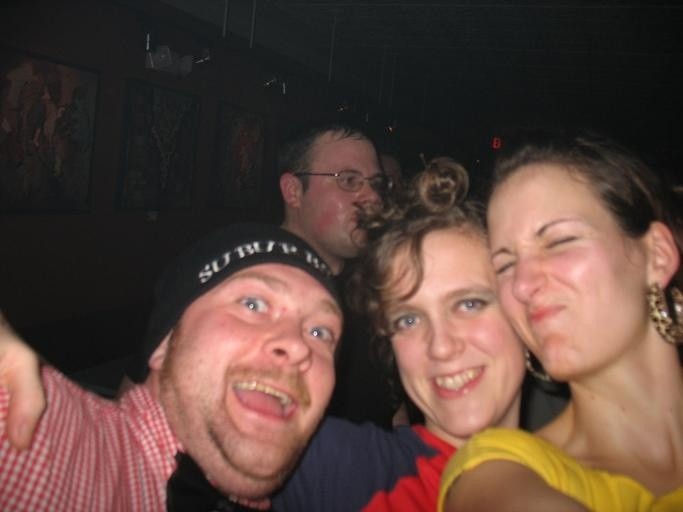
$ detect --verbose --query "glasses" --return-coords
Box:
[293,168,394,196]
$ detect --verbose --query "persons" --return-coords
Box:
[438,127,683,512]
[0,223,344,512]
[272,113,389,280]
[0,156,531,512]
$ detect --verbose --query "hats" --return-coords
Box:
[141,220,346,368]
